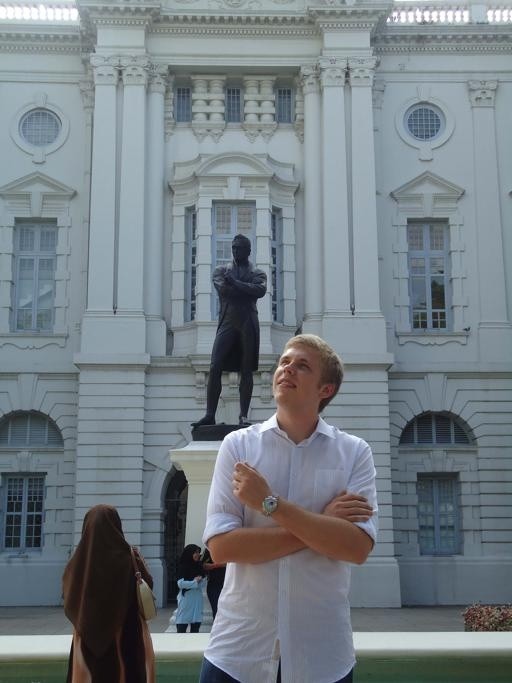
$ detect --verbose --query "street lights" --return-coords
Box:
[128,544,158,623]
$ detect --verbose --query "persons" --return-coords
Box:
[61,504,158,683]
[196,332,378,682]
[190,233,267,426]
[175,544,207,631]
[202,548,227,625]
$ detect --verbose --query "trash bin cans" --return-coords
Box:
[261,491,279,520]
